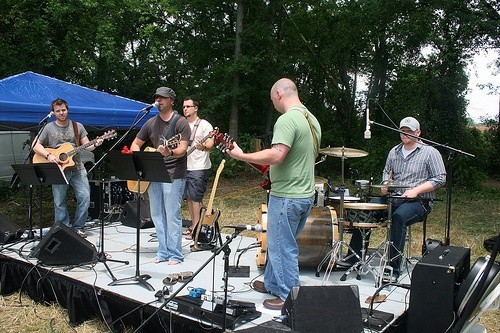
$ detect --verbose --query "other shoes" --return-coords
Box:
[182,228,193,240]
[382,273,398,283]
[335,259,352,268]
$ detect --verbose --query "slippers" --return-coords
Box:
[163,271,194,285]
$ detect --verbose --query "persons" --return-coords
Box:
[331,117,447,287]
[130,87,191,264]
[31,97,105,237]
[170,96,216,254]
[226,78,323,310]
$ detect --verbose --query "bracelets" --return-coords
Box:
[163,147,174,160]
[44,153,50,160]
[94,141,102,148]
[201,145,207,152]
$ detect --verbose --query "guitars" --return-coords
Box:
[32,128,118,172]
[126,132,181,194]
[191,158,226,244]
[214,132,271,190]
[186,126,219,157]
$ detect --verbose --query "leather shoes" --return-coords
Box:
[263,297,285,310]
[253,281,268,293]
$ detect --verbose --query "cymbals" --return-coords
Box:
[318,147,369,158]
[361,184,416,188]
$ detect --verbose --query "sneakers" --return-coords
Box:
[74,228,87,238]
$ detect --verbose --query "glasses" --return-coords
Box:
[155,96,167,99]
[182,106,196,109]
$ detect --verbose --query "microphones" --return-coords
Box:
[142,102,160,110]
[225,224,263,232]
[39,111,54,124]
[364,100,372,139]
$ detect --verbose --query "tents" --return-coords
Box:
[0,70,159,226]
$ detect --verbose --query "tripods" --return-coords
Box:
[63,149,175,292]
[0,121,47,251]
[315,151,415,285]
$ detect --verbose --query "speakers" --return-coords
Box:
[120,199,156,228]
[33,221,96,266]
[0,217,24,244]
[282,285,364,333]
[408,244,471,333]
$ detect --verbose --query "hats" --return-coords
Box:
[153,87,176,98]
[399,117,421,131]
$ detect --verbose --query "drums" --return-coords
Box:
[256,203,340,269]
[314,175,330,207]
[328,196,361,219]
[337,202,389,229]
